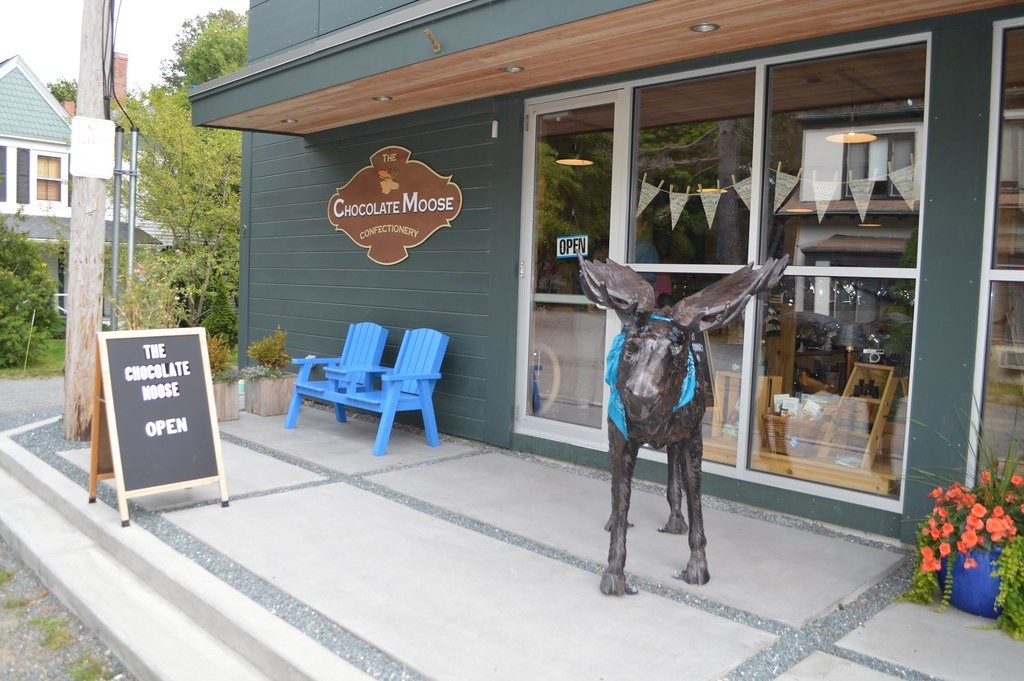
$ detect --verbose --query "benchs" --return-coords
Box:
[283,322,450,456]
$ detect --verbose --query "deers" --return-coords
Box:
[575,252,789,597]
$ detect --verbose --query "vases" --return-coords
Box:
[933,547,1006,621]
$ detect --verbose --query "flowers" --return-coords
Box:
[899,382,1023,640]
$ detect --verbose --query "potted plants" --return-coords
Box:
[241,327,297,418]
[207,329,240,423]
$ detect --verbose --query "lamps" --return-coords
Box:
[555,142,593,166]
[825,122,878,144]
